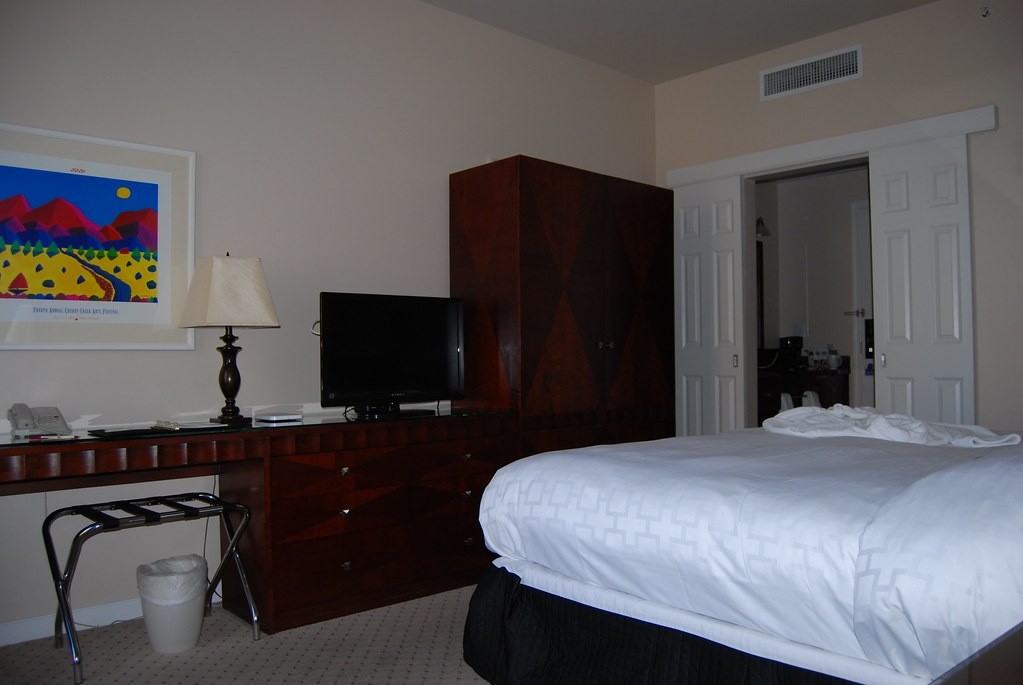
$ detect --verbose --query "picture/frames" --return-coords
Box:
[0,124,195,352]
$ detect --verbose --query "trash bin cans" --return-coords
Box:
[135,554,208,654]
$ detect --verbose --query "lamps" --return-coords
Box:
[176,251,281,422]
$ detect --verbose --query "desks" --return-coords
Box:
[758,365,849,424]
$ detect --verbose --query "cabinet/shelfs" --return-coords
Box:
[0,404,514,638]
[449,153,678,459]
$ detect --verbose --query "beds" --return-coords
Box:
[460,400,1023,684]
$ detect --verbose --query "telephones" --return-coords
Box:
[7,402,72,436]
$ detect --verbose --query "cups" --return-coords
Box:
[829,349,842,370]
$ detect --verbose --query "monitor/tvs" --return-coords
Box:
[319,292,465,422]
